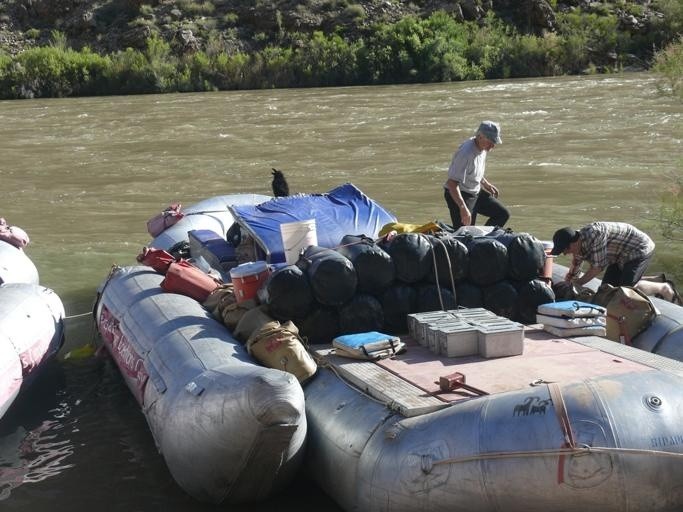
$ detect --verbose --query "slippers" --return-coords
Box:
[656,274,682,306]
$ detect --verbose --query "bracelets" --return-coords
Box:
[458,203,465,210]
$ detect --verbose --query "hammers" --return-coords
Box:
[440,372,490,396]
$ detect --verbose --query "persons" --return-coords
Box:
[442,119,509,232]
[548,218,681,306]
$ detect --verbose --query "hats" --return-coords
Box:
[480,121,503,145]
[551,227,575,255]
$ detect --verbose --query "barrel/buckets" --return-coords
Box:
[540,241,557,287]
[280,219,318,265]
[230,261,271,303]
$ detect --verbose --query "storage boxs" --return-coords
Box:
[406,306,524,359]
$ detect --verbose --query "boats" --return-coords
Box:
[0,216,68,424]
[90,186,681,511]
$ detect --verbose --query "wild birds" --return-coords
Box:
[269,167,290,198]
[225,222,243,248]
